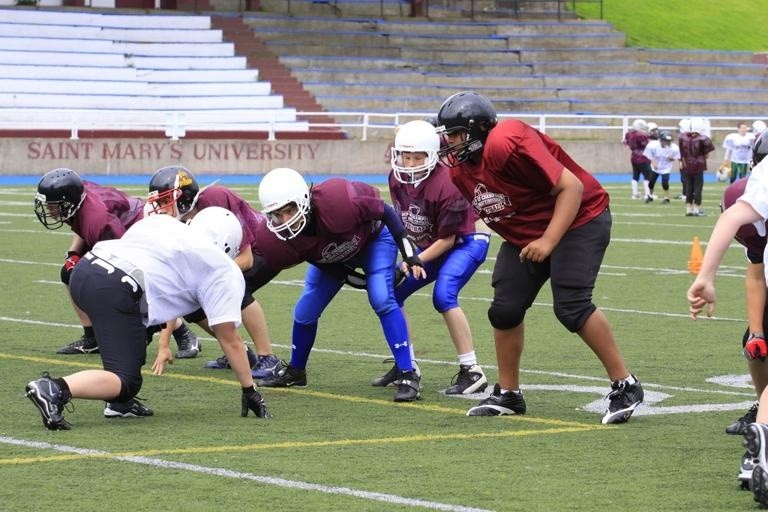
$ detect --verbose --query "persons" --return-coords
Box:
[372,120,491,395]
[34,168,203,359]
[148,166,282,378]
[25,205,275,430]
[726,128,768,435]
[625,117,766,217]
[435,91,644,424]
[686,154,768,506]
[256,168,427,402]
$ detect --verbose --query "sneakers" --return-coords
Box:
[725,402,759,435]
[632,192,640,200]
[250,353,283,379]
[204,345,258,370]
[174,325,202,359]
[370,357,405,388]
[393,370,421,403]
[255,359,309,388]
[734,418,768,506]
[443,363,489,397]
[102,395,155,418]
[685,210,692,217]
[673,194,683,200]
[600,375,645,426]
[693,209,706,216]
[56,333,101,354]
[466,382,527,418]
[663,197,670,204]
[23,371,76,431]
[645,194,658,203]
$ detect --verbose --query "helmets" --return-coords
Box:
[257,167,312,241]
[632,118,648,132]
[660,131,674,146]
[186,206,244,261]
[717,164,732,183]
[678,117,689,132]
[147,165,200,218]
[648,120,658,138]
[390,119,441,185]
[752,133,768,164]
[752,120,766,132]
[437,91,499,165]
[692,119,705,133]
[34,167,87,230]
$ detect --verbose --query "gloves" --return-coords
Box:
[60,248,80,286]
[744,334,768,363]
[240,383,273,420]
